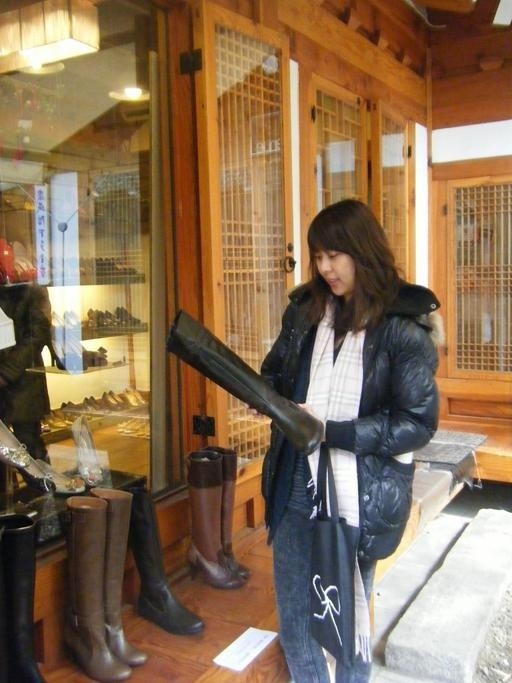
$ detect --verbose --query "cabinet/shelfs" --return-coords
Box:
[41,272,148,448]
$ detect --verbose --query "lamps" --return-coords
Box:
[109,64,153,101]
[0,0,101,72]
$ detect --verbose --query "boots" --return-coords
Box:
[205,445,249,581]
[0,515,48,682]
[186,451,247,589]
[63,495,132,683]
[119,476,204,634]
[89,487,147,666]
[167,309,325,454]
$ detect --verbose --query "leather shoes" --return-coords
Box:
[17,454,55,495]
[81,257,138,277]
[87,307,142,328]
[0,420,30,468]
[39,388,147,433]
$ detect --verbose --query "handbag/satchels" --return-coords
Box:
[308,443,363,668]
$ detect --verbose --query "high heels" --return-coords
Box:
[71,414,105,488]
[36,458,85,494]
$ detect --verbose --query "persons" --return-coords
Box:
[261,201,439,683]
[0,283,52,488]
